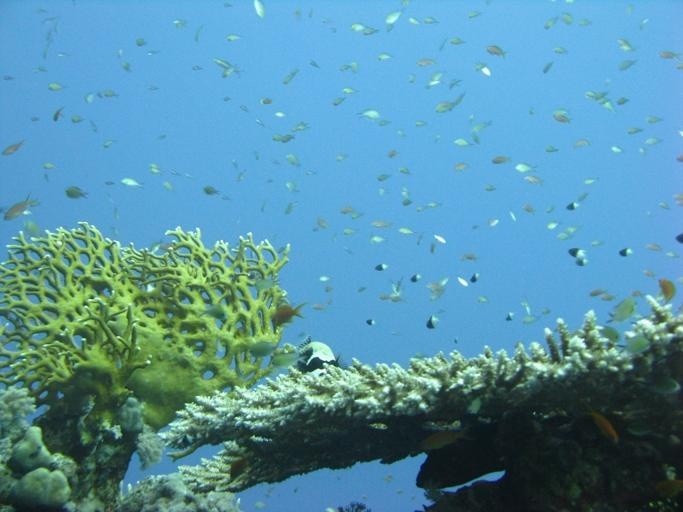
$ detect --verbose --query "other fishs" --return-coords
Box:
[1,0,682,331]
